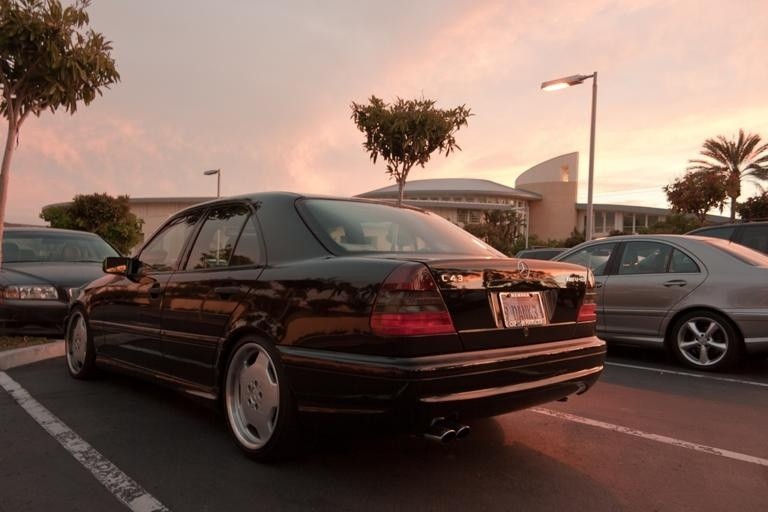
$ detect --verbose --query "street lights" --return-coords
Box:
[539,70,600,242]
[204,168,221,200]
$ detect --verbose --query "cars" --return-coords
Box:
[55,190,610,465]
[515,218,768,374]
[1,224,123,341]
